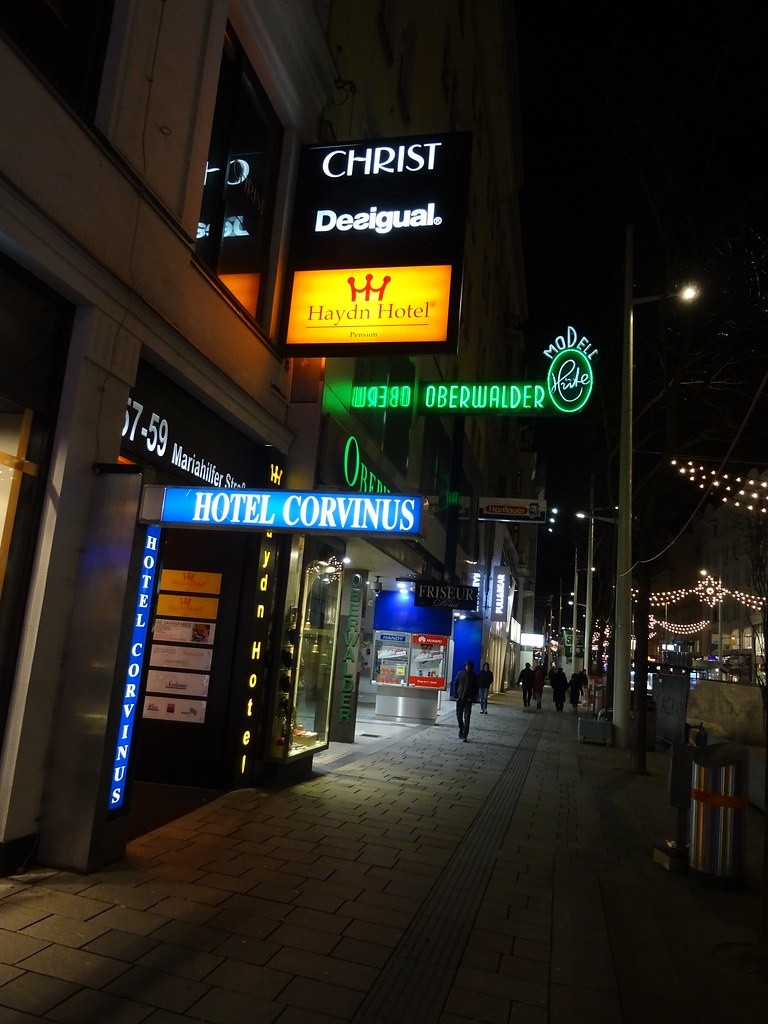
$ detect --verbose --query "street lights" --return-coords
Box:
[610,207,700,746]
[551,470,639,697]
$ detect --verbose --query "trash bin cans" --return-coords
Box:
[687,743,748,876]
[632,698,657,751]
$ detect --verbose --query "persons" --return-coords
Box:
[548,667,556,688]
[551,668,568,712]
[518,663,534,707]
[578,669,587,685]
[453,660,480,742]
[568,673,584,714]
[533,666,545,709]
[477,663,493,714]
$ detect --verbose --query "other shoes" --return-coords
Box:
[480,712,483,714]
[485,712,487,714]
[463,738,467,742]
[459,731,462,738]
